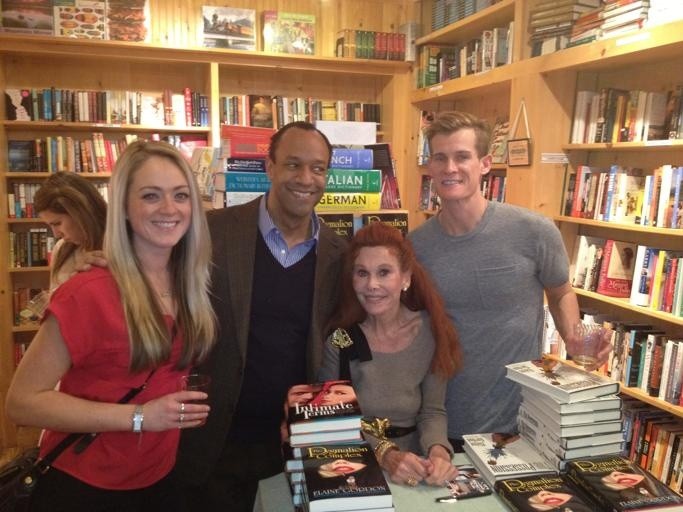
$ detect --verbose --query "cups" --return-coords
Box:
[176,373,211,429]
[573,323,605,369]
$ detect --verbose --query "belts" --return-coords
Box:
[382,425,420,439]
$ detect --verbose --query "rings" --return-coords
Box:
[407,478,416,485]
[179,402,184,412]
[178,413,186,422]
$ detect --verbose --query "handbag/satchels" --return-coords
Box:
[0,445,45,512]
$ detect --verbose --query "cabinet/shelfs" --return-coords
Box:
[412,2,680,505]
[0,2,410,453]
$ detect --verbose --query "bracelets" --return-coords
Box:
[132,404,145,432]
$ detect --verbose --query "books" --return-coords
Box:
[562,87,683,229]
[413,0,681,90]
[457,353,681,511]
[543,234,681,405]
[415,107,514,210]
[12,287,47,369]
[269,379,396,511]
[0,0,417,63]
[6,180,114,268]
[190,93,410,241]
[3,86,211,175]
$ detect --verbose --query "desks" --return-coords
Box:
[258,450,675,509]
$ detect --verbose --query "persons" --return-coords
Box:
[67,121,348,512]
[403,111,613,453]
[5,138,223,510]
[31,170,110,286]
[317,223,463,489]
